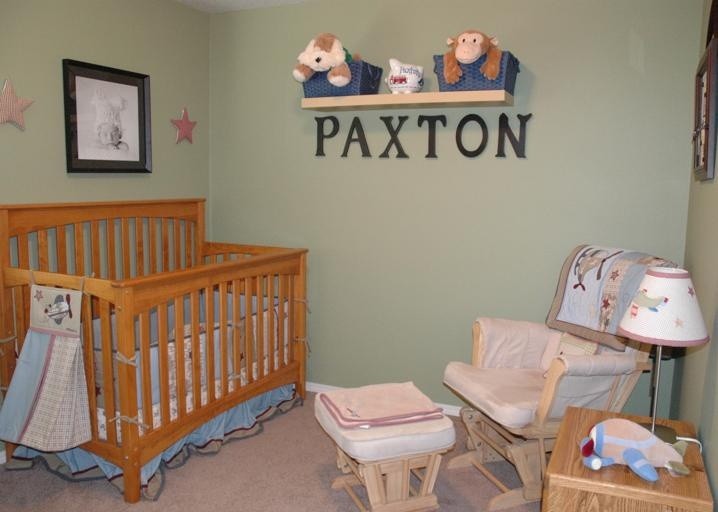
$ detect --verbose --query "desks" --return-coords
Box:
[541,405,718,512]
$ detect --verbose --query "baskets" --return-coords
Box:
[433,51,520,96]
[302,60,382,98]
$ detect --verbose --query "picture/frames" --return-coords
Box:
[690,34,716,183]
[62,57,152,176]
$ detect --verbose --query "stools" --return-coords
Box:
[311,382,455,511]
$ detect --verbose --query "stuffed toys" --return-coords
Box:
[441,29,504,84]
[290,30,362,88]
[576,417,688,483]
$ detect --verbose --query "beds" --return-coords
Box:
[3,197,307,503]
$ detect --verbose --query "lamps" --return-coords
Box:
[618,265,711,445]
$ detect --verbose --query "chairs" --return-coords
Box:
[444,247,680,506]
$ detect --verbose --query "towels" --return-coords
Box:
[319,378,443,432]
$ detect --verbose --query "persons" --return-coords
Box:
[89,89,131,155]
